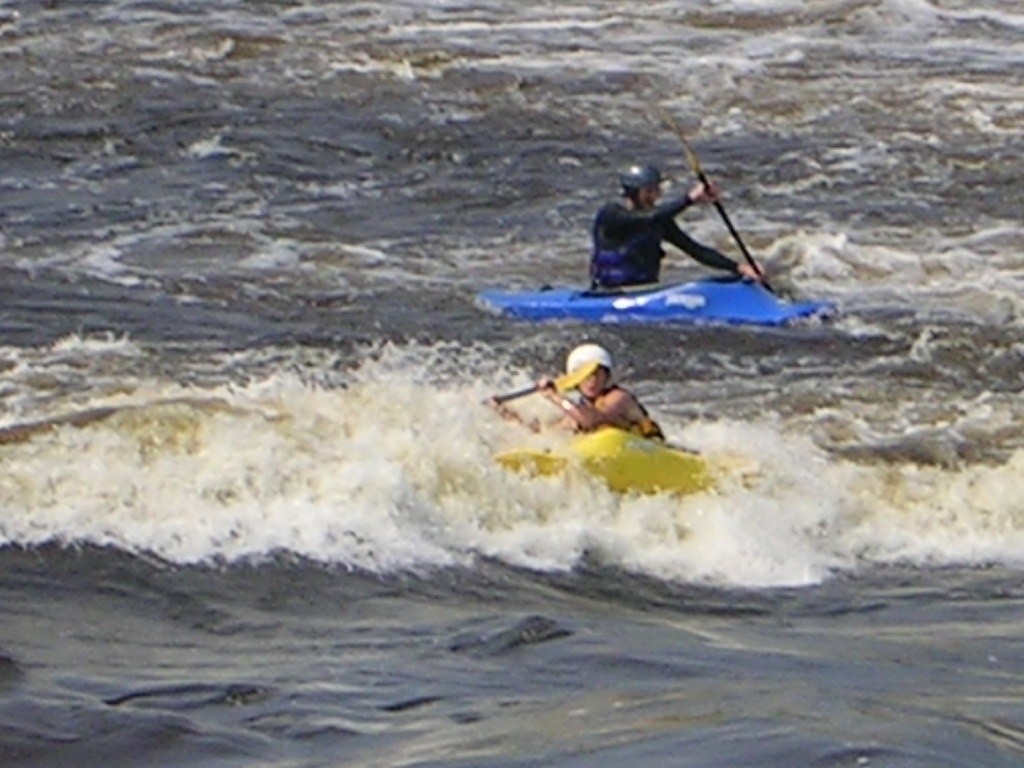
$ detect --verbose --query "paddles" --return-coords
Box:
[477,358,604,405]
[658,102,775,294]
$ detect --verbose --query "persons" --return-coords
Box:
[589,163,766,288]
[488,344,664,444]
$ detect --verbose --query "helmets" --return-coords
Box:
[618,160,662,196]
[566,343,613,376]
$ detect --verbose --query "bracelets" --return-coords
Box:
[562,399,576,413]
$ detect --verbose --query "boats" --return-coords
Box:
[477,272,845,330]
[489,425,723,501]
